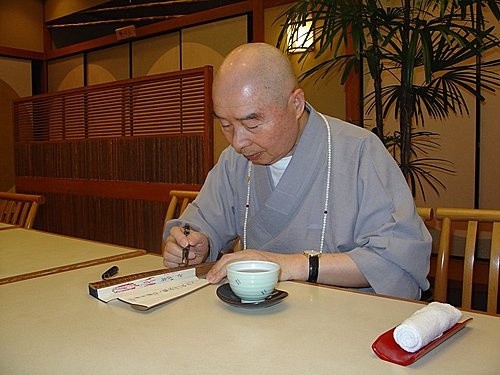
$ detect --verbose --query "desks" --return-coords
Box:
[0,253,500,375]
[1,222,147,284]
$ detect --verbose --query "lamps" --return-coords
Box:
[287,13,315,54]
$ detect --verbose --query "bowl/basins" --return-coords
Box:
[225,260,280,303]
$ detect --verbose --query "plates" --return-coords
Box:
[216,282,289,309]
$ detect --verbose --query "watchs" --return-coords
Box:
[303,248,321,283]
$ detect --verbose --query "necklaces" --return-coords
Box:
[243,111,331,253]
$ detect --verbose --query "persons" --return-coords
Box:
[164,42,432,301]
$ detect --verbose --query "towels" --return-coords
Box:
[392,300,464,354]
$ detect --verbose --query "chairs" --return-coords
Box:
[0,191,45,229]
[161,190,241,261]
[436,207,500,318]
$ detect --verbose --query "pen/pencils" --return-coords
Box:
[181,222,190,264]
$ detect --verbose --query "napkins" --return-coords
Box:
[394,302,462,353]
